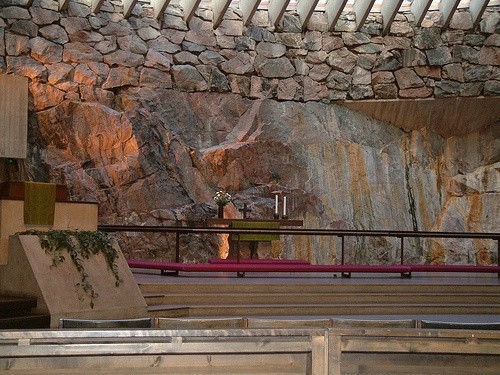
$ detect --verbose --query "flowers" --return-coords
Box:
[213,191,232,206]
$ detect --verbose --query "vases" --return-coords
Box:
[218,204,224,219]
